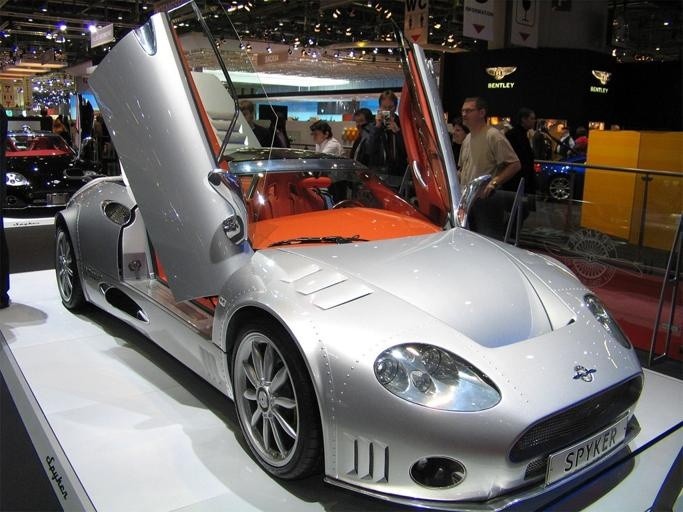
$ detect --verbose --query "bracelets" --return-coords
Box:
[490,178,499,188]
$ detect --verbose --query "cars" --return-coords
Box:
[1,129,96,217]
[532,155,586,203]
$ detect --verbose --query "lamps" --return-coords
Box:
[213,0,463,62]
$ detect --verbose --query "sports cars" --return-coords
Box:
[53,1,644,512]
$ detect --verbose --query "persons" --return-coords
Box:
[264,111,292,149]
[458,98,521,237]
[371,92,408,173]
[347,107,374,166]
[238,100,273,149]
[437,105,622,241]
[1,99,114,308]
[306,119,347,199]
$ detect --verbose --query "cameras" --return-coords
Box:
[376,110,390,120]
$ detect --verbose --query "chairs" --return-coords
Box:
[29,137,58,150]
[6,137,19,151]
[260,173,315,221]
